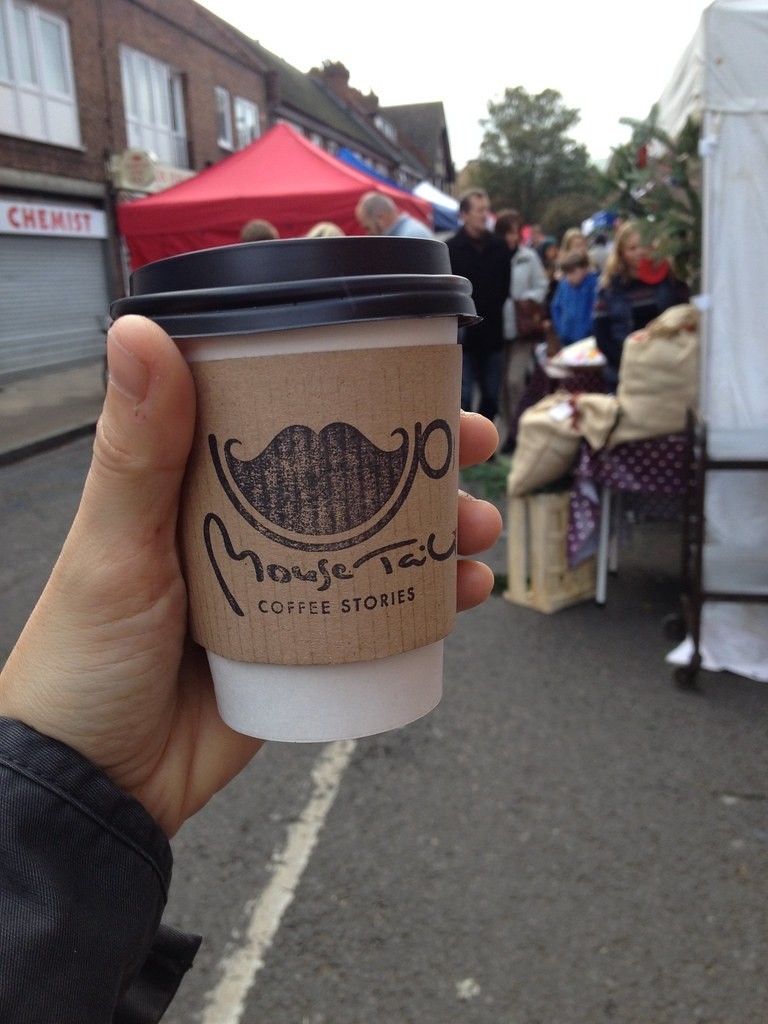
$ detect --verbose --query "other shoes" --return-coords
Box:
[500,433,515,454]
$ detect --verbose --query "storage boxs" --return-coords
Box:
[504,493,597,615]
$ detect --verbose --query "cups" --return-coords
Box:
[109,237,477,743]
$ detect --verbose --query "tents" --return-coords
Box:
[336,148,457,229]
[414,181,495,231]
[116,124,433,271]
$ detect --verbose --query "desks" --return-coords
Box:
[566,434,699,605]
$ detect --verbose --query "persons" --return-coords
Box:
[305,222,345,237]
[445,188,692,463]
[240,219,280,241]
[356,193,434,240]
[0,313,503,1024]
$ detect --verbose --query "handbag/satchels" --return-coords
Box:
[513,299,551,343]
